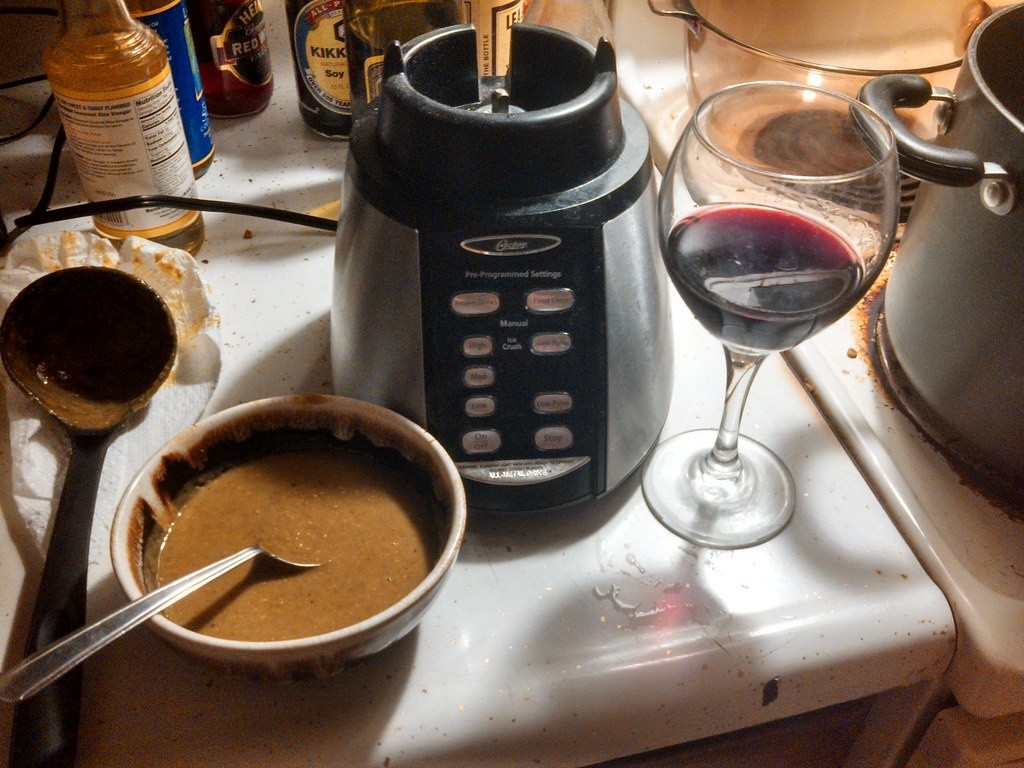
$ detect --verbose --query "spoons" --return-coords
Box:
[0,535,316,706]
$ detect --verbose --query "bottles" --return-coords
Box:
[121,0,216,180]
[42,0,206,264]
[340,1,469,151]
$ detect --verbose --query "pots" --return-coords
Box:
[854,14,1021,503]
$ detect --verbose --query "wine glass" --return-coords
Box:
[633,79,901,553]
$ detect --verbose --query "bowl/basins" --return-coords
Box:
[109,394,471,677]
[645,1,995,182]
